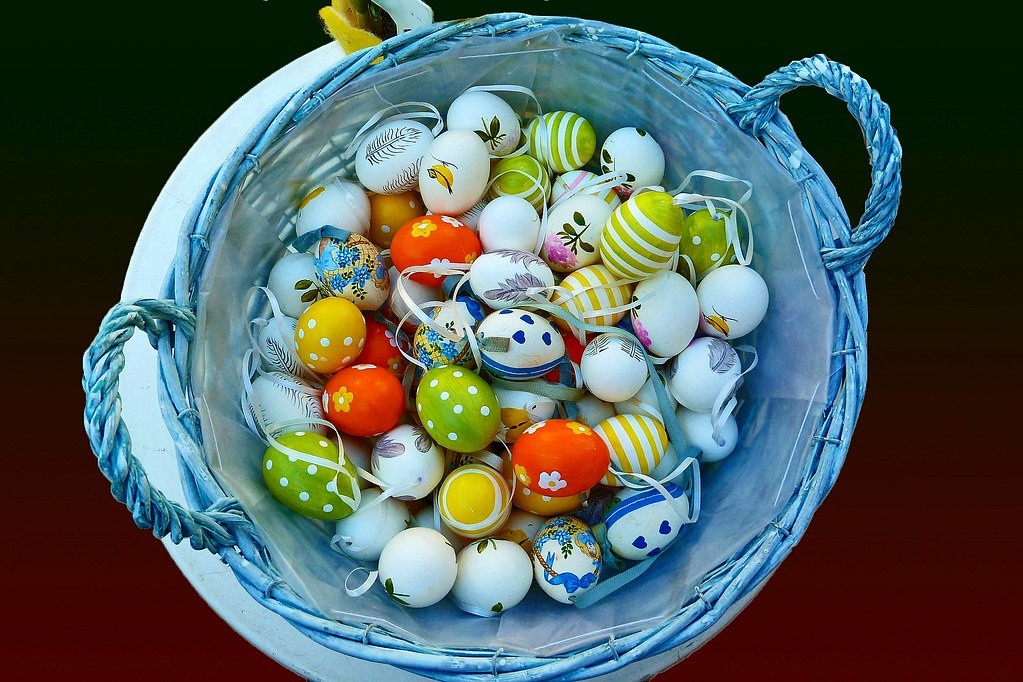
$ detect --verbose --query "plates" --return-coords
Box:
[107,19,820,681]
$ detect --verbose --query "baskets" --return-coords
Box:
[79,12,904,682]
